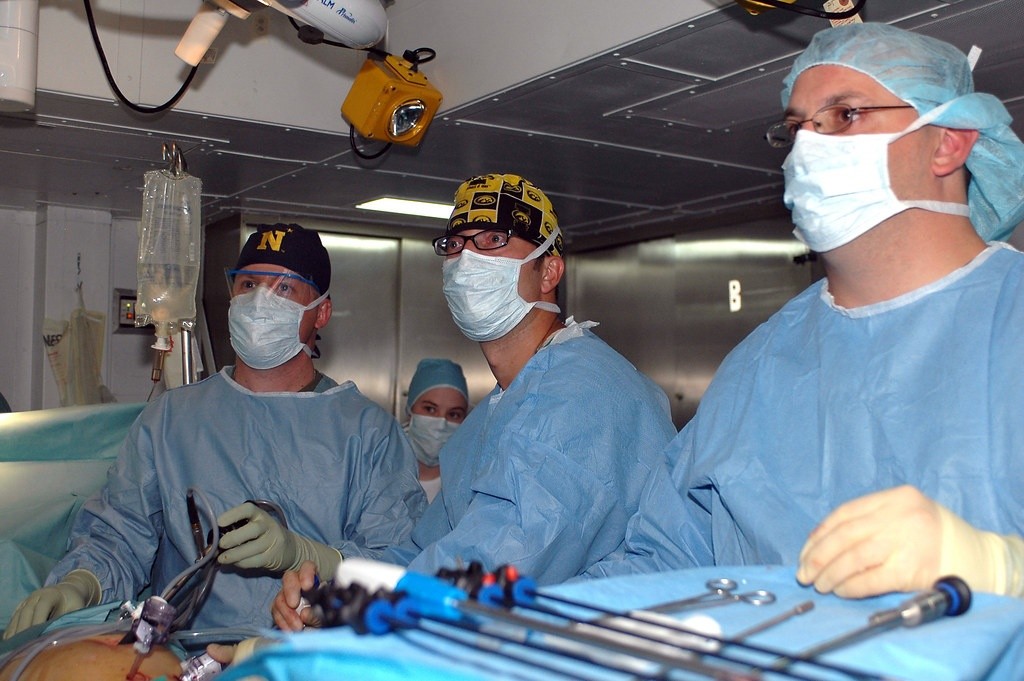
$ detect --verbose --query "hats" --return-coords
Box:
[446,172,563,258]
[231,223,330,300]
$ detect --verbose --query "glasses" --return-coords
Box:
[763,103,913,149]
[432,229,550,257]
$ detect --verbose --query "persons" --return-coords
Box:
[1,624,185,681]
[550,23,1024,600]
[3,222,429,660]
[271,173,680,639]
[401,359,468,506]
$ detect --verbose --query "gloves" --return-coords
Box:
[797,485,1024,599]
[216,503,342,586]
[2,569,102,639]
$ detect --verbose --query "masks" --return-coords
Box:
[781,100,970,252]
[404,411,461,468]
[227,288,330,370]
[441,230,561,342]
